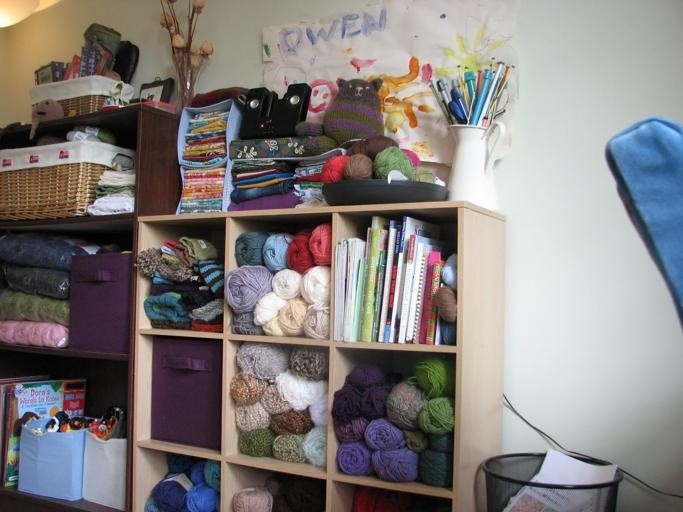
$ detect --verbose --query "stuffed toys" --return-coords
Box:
[28,99,64,141]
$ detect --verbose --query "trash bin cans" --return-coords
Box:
[480,454,624,512]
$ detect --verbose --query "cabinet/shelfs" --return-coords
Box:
[128,200,507,511]
[0,102,183,512]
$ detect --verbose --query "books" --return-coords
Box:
[0,372,86,490]
[331,212,441,348]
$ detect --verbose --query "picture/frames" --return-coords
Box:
[138,76,175,103]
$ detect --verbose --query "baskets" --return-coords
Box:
[0,141,135,220]
[28,74,132,123]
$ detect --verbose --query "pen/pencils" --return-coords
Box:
[429,56,515,127]
